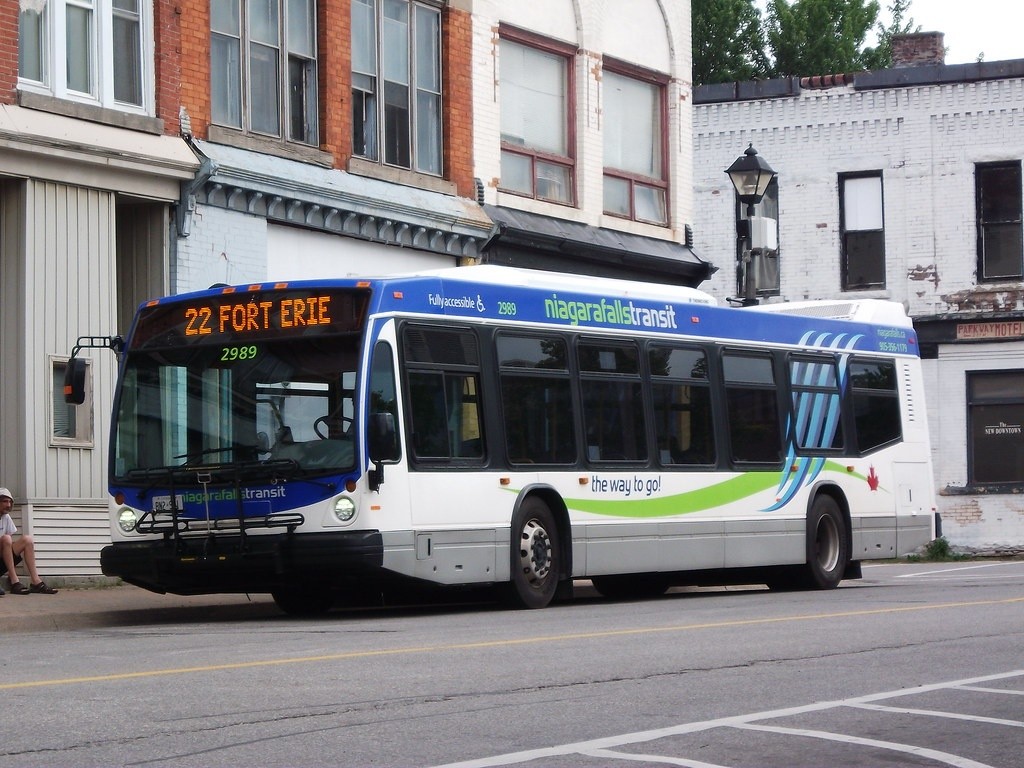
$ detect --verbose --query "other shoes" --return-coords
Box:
[10,582,31,594]
[29,581,58,594]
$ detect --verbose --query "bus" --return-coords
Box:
[63,263,944,619]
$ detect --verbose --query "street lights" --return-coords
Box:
[723,144,779,309]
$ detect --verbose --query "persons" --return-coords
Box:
[350,376,397,457]
[0,487,58,594]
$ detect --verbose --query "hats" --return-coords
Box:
[1,487,14,502]
